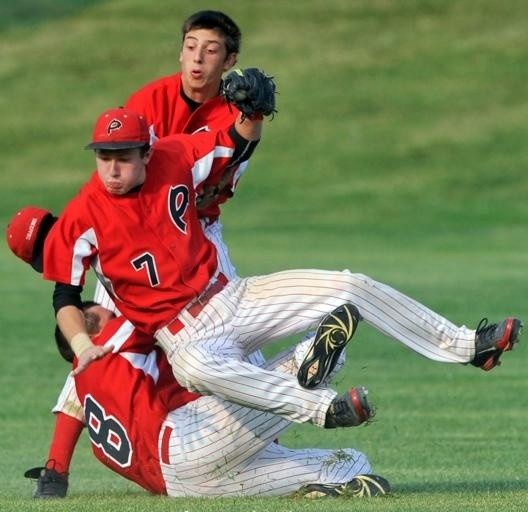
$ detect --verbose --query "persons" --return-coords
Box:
[40,67,524,431]
[22,9,241,504]
[51,301,391,502]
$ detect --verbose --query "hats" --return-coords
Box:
[83,105,151,150]
[5,206,61,273]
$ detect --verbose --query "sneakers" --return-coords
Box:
[298,474,392,502]
[329,383,372,428]
[295,303,360,390]
[23,457,71,500]
[467,316,525,372]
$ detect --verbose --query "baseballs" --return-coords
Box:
[234,90,247,100]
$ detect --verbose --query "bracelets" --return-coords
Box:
[70,333,96,358]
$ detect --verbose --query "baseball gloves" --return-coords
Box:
[219,68,280,126]
